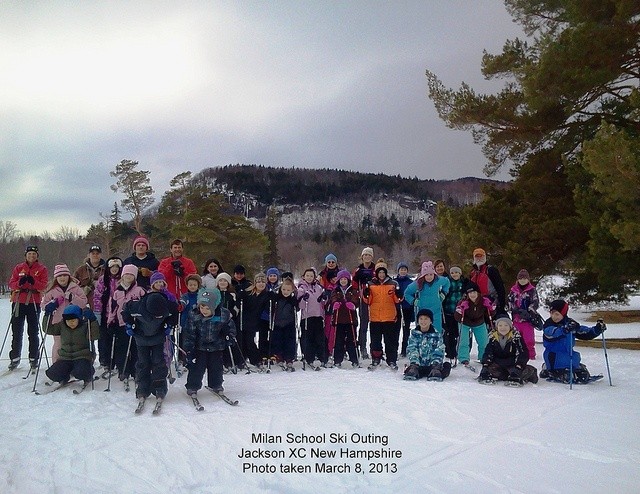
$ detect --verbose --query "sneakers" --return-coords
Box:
[136,385,151,398]
[59,377,70,385]
[286,362,293,367]
[540,362,549,378]
[150,382,167,396]
[270,355,280,364]
[187,388,197,395]
[368,360,383,370]
[257,361,265,370]
[262,356,268,364]
[351,362,361,368]
[84,366,95,381]
[8,357,21,368]
[208,384,224,394]
[461,360,469,366]
[331,363,341,368]
[30,358,38,368]
[277,359,284,366]
[386,360,398,370]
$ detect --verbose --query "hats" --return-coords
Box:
[494,313,512,329]
[24,246,39,258]
[517,269,530,279]
[374,258,387,278]
[473,248,486,256]
[550,297,568,318]
[418,260,438,280]
[150,270,168,290]
[325,253,337,265]
[89,244,101,253]
[253,273,267,283]
[184,274,202,287]
[146,294,166,315]
[121,264,139,280]
[335,270,351,283]
[267,268,279,278]
[53,264,70,277]
[132,235,149,251]
[466,282,479,293]
[234,264,245,273]
[281,271,293,281]
[450,263,462,276]
[215,272,231,286]
[361,247,374,258]
[197,286,221,315]
[397,261,408,272]
[62,305,82,325]
[107,257,123,267]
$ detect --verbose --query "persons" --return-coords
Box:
[455,280,497,364]
[273,280,301,367]
[93,257,124,374]
[539,299,606,383]
[147,270,179,375]
[404,260,451,338]
[71,244,106,365]
[325,270,340,357]
[297,268,329,367]
[178,272,202,373]
[392,262,415,355]
[123,236,160,291]
[107,264,145,381]
[216,272,245,371]
[506,268,540,359]
[121,291,177,398]
[201,258,224,288]
[363,258,404,370]
[351,247,375,360]
[469,248,507,332]
[42,301,100,384]
[41,262,89,365]
[403,308,445,380]
[242,271,272,365]
[331,268,360,368]
[433,259,449,277]
[480,311,529,383]
[8,245,48,367]
[281,270,298,295]
[315,252,342,289]
[231,264,253,296]
[157,239,198,311]
[444,263,472,356]
[265,268,281,291]
[184,285,236,395]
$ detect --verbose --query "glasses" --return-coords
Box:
[26,247,38,251]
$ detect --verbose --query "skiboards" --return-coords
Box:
[0,367,36,378]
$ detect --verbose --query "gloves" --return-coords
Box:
[237,288,247,300]
[82,307,96,322]
[413,299,418,306]
[513,307,522,314]
[108,317,119,332]
[93,311,102,325]
[395,289,404,299]
[317,292,327,303]
[80,286,91,295]
[140,267,154,278]
[528,304,537,313]
[159,285,175,302]
[291,297,298,305]
[428,364,443,376]
[439,292,444,301]
[456,301,469,314]
[363,287,371,299]
[510,366,522,381]
[480,366,493,383]
[65,292,72,299]
[56,296,64,307]
[303,293,309,300]
[184,354,193,365]
[564,320,579,333]
[170,259,182,267]
[19,276,26,285]
[482,296,496,311]
[406,363,420,377]
[333,301,342,310]
[596,322,606,332]
[173,266,184,276]
[26,274,34,283]
[177,304,184,312]
[45,303,58,316]
[227,337,237,347]
[125,322,135,336]
[162,322,172,336]
[345,302,356,311]
[92,280,98,288]
